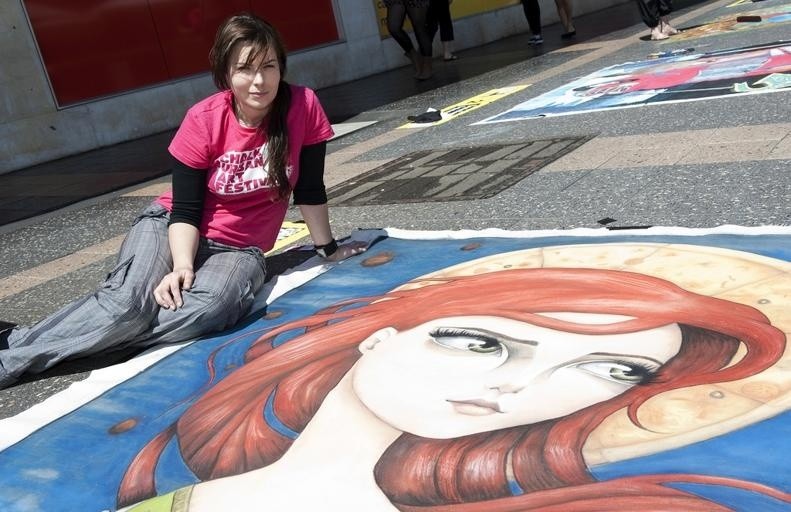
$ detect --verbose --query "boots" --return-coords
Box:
[403,51,433,80]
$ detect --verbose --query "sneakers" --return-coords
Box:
[560,24,576,36]
[528,35,544,45]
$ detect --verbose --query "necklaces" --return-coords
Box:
[236,105,269,127]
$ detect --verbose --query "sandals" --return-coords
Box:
[651,28,677,40]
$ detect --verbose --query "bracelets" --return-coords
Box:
[312,238,338,260]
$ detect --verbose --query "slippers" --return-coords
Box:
[444,53,455,59]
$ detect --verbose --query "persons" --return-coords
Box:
[521,0,545,45]
[637,0,685,42]
[385,1,441,82]
[557,1,578,39]
[106,236,790,512]
[2,13,368,393]
[439,1,460,62]
